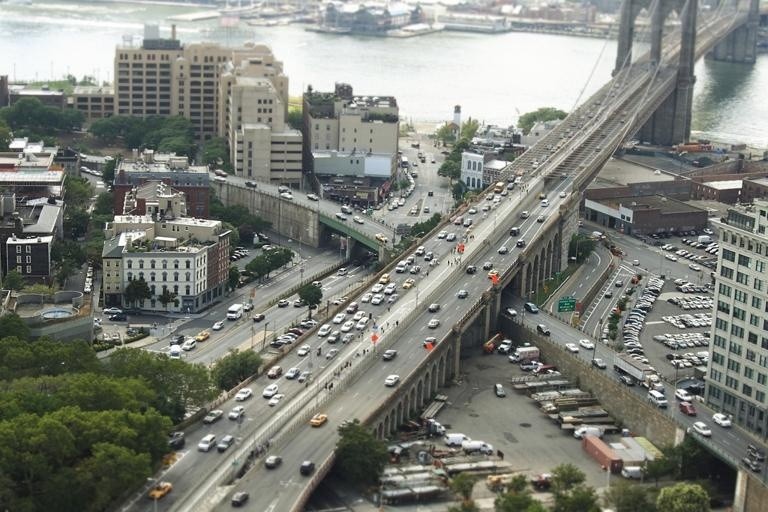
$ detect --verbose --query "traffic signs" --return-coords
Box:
[557,299,576,314]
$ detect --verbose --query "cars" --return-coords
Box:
[103,307,127,321]
[83,266,94,293]
[565,85,629,170]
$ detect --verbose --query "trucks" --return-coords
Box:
[670,141,702,156]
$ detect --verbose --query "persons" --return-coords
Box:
[323,317,399,391]
[226,438,275,484]
[426,271,429,277]
[387,303,390,312]
[519,183,530,193]
[447,231,475,268]
